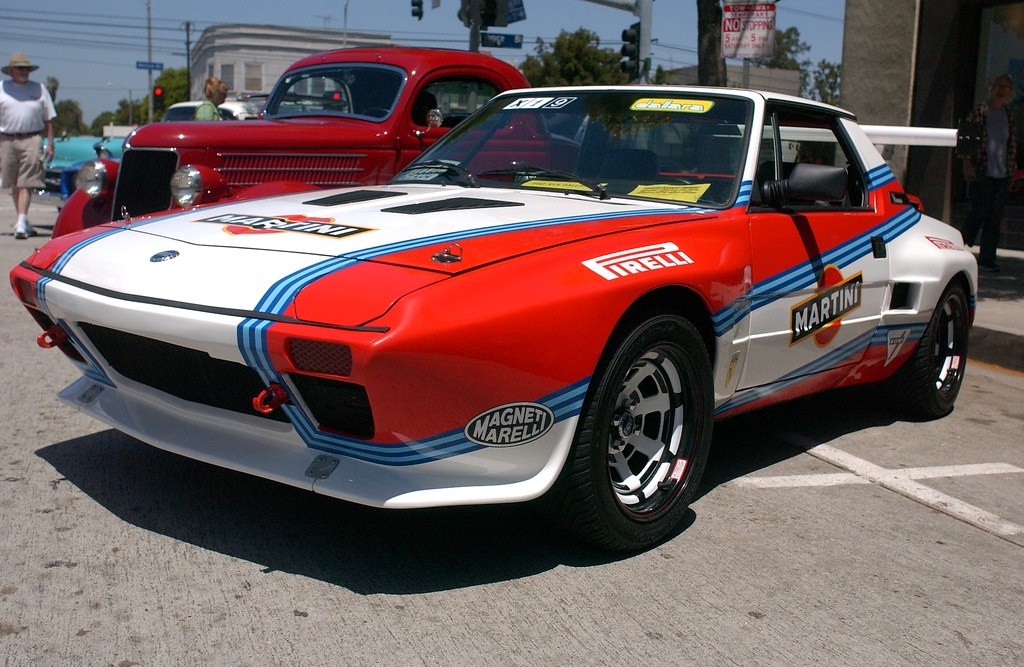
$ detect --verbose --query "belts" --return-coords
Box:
[1,131,40,137]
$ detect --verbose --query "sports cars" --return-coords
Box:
[8,83,983,555]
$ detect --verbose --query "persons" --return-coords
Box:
[955,73,1017,272]
[0,52,57,239]
[194,77,229,121]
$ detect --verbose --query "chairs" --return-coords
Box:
[411,92,437,125]
[760,162,814,205]
[601,149,660,181]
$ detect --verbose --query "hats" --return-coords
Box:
[1,52,40,74]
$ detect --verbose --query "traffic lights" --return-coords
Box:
[621,21,642,82]
[409,0,425,20]
[153,87,165,110]
[327,90,342,113]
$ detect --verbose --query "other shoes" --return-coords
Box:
[14,225,28,239]
[977,257,1000,272]
[26,227,36,237]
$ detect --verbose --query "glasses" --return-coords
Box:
[993,84,1013,92]
[17,66,31,71]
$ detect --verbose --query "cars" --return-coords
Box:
[49,43,575,252]
[39,90,326,205]
